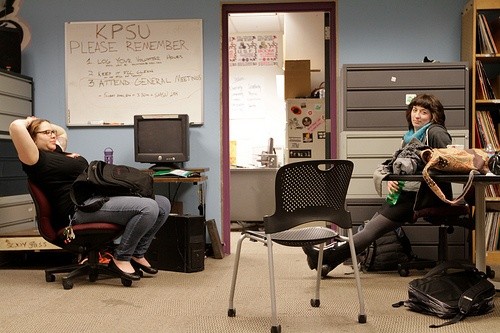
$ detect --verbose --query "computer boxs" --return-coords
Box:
[147,213,205,273]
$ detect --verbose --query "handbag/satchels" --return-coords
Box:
[422,148,489,206]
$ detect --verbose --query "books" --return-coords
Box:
[485,184,500,197]
[475,111,499,148]
[485,211,500,251]
[476,13,498,54]
[476,60,496,99]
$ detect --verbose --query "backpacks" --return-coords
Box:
[69,160,153,212]
[358,219,416,273]
[392,263,495,328]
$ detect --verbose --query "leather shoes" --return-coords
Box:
[131,258,158,274]
[108,259,141,280]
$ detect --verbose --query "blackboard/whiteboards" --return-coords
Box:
[63,19,204,128]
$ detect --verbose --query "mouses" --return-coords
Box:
[187,171,201,178]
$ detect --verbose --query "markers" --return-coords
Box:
[103,122,124,126]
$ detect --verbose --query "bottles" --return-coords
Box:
[103,146,114,165]
[483,143,496,173]
[385,180,404,207]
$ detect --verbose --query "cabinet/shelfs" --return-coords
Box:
[462,0,500,269]
[340,62,469,268]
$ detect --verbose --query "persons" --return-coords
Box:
[8,116,171,281]
[302,93,452,276]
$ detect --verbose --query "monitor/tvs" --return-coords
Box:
[134,114,190,170]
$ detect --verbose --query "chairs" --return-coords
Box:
[26,182,132,289]
[228,158,367,333]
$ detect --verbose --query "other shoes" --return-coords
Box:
[303,246,333,278]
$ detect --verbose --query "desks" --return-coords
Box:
[140,168,208,259]
[374,172,500,273]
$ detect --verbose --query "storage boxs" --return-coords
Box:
[284,59,311,99]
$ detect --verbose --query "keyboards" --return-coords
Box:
[154,174,181,178]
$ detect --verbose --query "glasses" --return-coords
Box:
[37,130,57,136]
